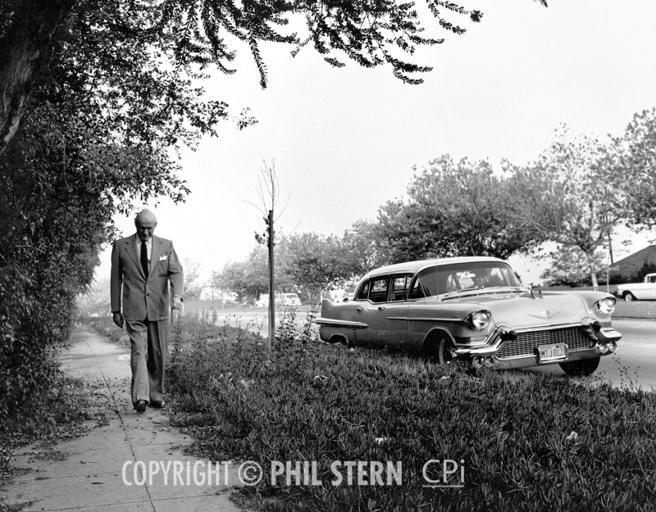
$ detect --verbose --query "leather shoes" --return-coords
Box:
[133,398,145,412]
[150,394,168,409]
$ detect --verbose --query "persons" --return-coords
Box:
[107,208,183,410]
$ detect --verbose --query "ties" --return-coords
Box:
[140,239,148,280]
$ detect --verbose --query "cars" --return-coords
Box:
[616,272,656,301]
[255,292,301,309]
[314,256,621,376]
